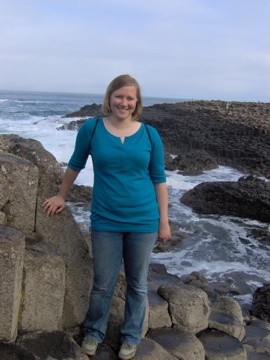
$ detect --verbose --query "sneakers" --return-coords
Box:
[81,336,98,355]
[118,345,137,359]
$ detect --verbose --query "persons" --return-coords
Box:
[42,74,172,360]
